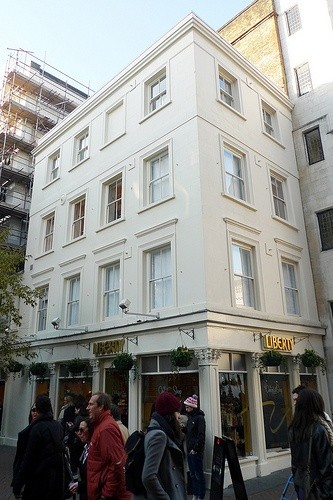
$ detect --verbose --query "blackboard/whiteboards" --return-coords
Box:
[210,435,247,500]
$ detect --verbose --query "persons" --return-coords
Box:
[288,384,333,500]
[11,390,207,500]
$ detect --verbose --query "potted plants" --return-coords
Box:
[170,347,193,368]
[109,353,140,382]
[295,349,329,376]
[259,351,283,366]
[5,361,26,382]
[27,361,52,384]
[61,359,92,378]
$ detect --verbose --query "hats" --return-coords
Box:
[183,395,198,408]
[155,391,183,414]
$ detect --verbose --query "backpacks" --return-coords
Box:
[125,428,169,496]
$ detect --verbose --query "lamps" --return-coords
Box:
[119,299,160,319]
[51,318,88,333]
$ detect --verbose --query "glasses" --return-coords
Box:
[75,428,84,433]
[32,408,36,412]
